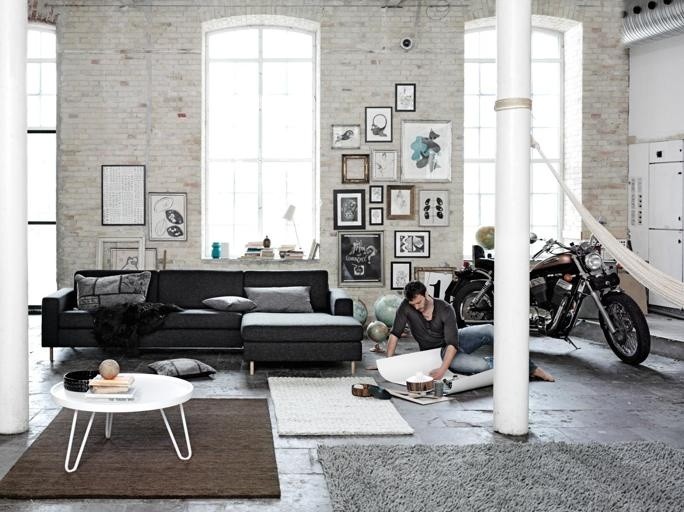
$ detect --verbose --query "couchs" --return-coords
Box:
[42,270,364,375]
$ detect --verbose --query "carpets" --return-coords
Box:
[0,398,280,499]
[317,440,684,512]
[266,375,415,437]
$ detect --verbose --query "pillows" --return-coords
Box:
[159,269,244,306]
[202,295,257,312]
[245,270,330,313]
[148,357,216,378]
[74,270,158,305]
[74,270,151,310]
[244,286,314,313]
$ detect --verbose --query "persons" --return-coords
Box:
[387,281,555,383]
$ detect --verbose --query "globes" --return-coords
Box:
[366,320,388,352]
[353,299,368,327]
[374,293,406,337]
[475,226,494,250]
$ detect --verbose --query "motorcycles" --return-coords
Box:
[443,216,652,368]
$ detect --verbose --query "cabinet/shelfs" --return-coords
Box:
[628,138,683,310]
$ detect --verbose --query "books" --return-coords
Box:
[85,375,136,399]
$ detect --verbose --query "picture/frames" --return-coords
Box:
[96,236,146,271]
[101,165,147,226]
[149,192,187,242]
[330,82,453,291]
